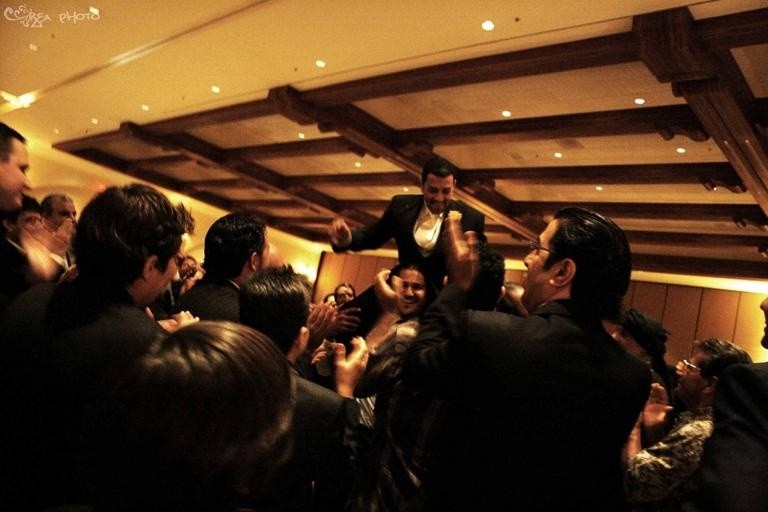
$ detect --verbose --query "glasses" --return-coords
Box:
[176,252,185,268]
[528,238,566,260]
[682,359,719,383]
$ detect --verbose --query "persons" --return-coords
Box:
[1,120,768,509]
